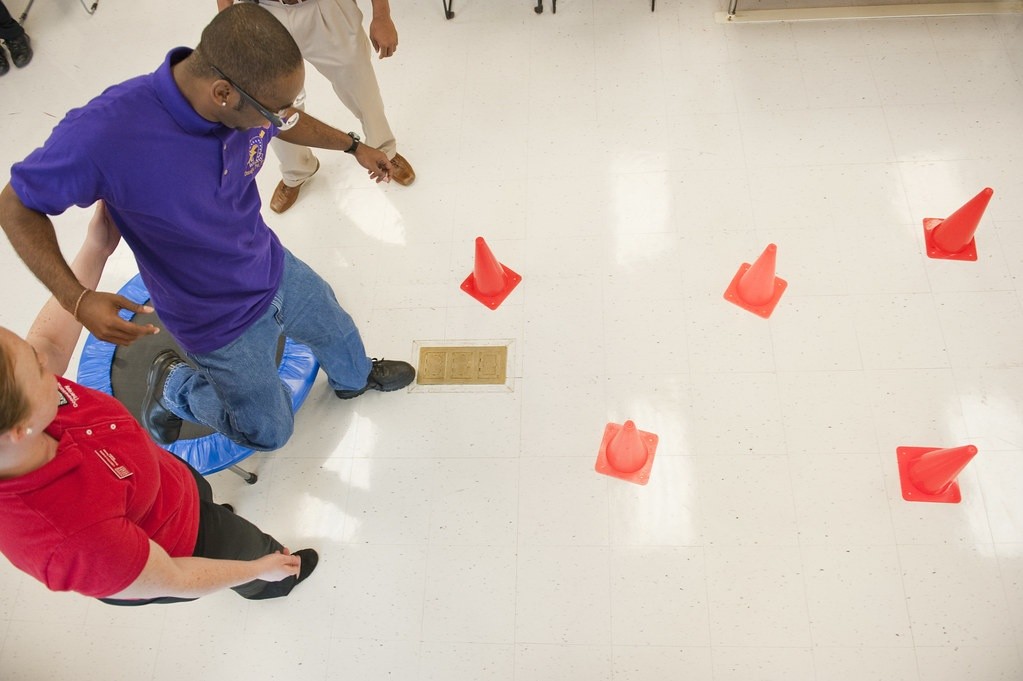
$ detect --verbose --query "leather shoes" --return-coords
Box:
[139,349,183,445]
[270,156,320,214]
[388,152,416,187]
[334,357,415,400]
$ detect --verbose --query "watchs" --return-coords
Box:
[344,132,360,153]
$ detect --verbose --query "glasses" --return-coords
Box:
[209,64,310,131]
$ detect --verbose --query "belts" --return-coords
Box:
[269,0,308,5]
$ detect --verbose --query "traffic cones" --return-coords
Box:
[594,419,659,486]
[460,235,523,311]
[722,242,788,320]
[922,187,994,262]
[897,445,978,504]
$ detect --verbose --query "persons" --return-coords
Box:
[216,0,416,214]
[0,2,416,451]
[0,197,318,606]
[0,0,33,76]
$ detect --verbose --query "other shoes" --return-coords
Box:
[284,548,318,586]
[0,46,9,76]
[220,503,233,512]
[4,31,33,68]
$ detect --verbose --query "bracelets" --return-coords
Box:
[74,290,89,321]
